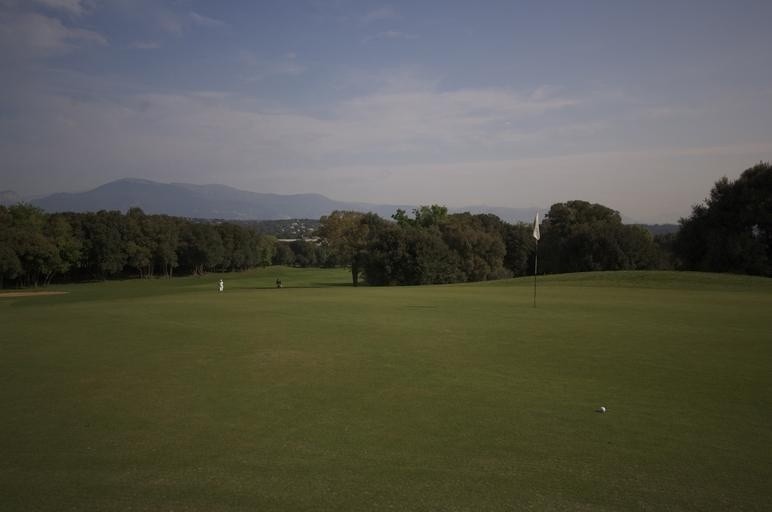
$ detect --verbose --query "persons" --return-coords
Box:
[277,277,281,288]
[216,279,224,296]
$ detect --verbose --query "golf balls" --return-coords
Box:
[601,407,606,412]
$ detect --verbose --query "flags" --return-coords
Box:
[532,212,540,240]
